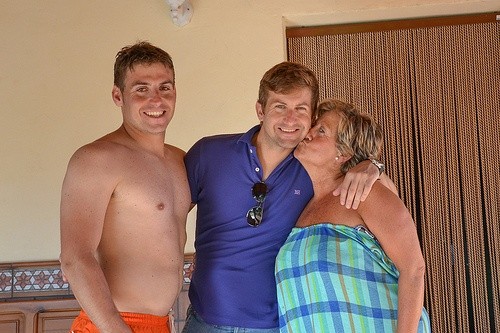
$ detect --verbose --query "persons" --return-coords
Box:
[274,99,430,333]
[183,61,399,333]
[59,41,188,333]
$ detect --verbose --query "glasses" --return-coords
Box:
[246,181,267,228]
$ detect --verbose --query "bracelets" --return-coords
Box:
[367,158,385,177]
